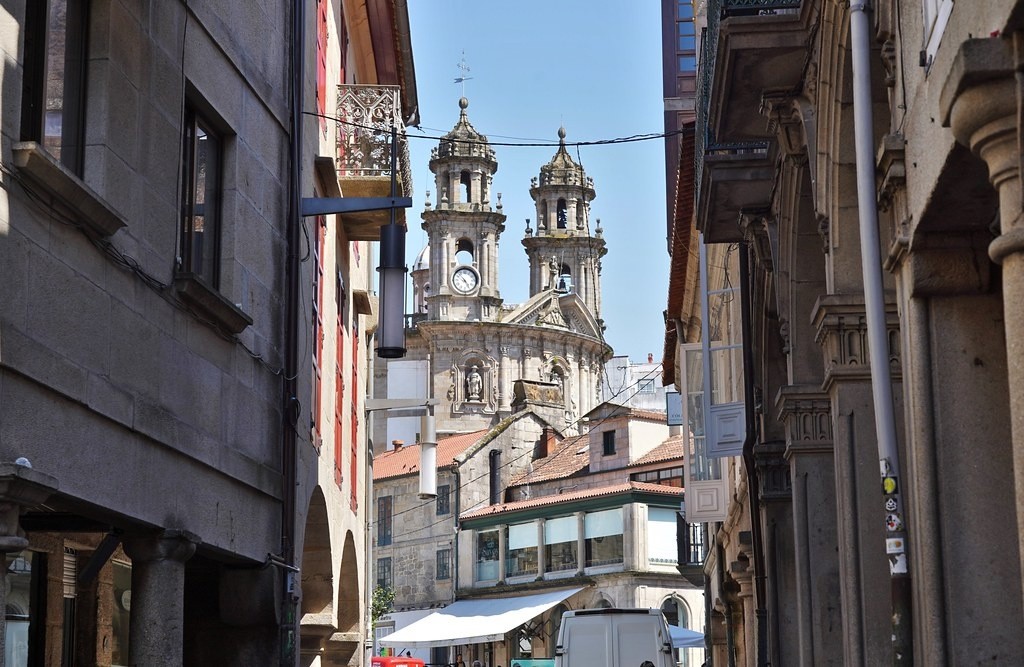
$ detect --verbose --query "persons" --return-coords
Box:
[406,650,413,658]
[551,373,559,383]
[454,654,466,667]
[464,364,483,396]
[471,656,483,667]
[548,255,563,290]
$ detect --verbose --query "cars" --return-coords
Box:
[371,657,425,667]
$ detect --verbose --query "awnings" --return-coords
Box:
[377,584,592,648]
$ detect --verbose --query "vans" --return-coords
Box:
[553,608,677,666]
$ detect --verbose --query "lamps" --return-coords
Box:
[420,415,438,500]
[374,223,408,357]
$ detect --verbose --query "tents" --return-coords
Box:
[669,624,705,648]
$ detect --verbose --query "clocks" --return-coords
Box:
[453,267,477,293]
